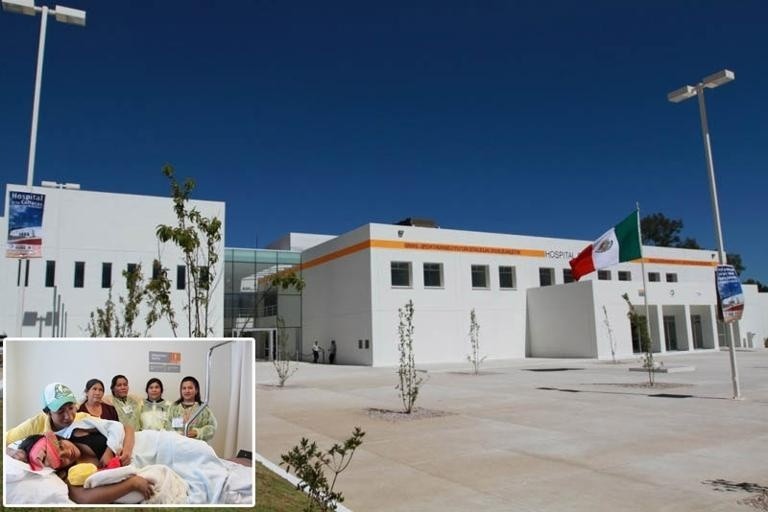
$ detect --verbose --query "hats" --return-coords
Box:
[40,382,77,412]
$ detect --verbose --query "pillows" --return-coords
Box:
[6,438,56,485]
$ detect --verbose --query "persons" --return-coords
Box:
[77,380,120,423]
[20,430,251,505]
[138,378,174,430]
[6,383,135,468]
[103,375,143,432]
[167,376,218,442]
[327,340,337,364]
[310,340,321,363]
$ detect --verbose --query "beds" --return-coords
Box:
[5,458,252,504]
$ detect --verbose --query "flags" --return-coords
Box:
[568,210,642,282]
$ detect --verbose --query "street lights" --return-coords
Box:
[666,69,741,397]
[1,0,86,337]
